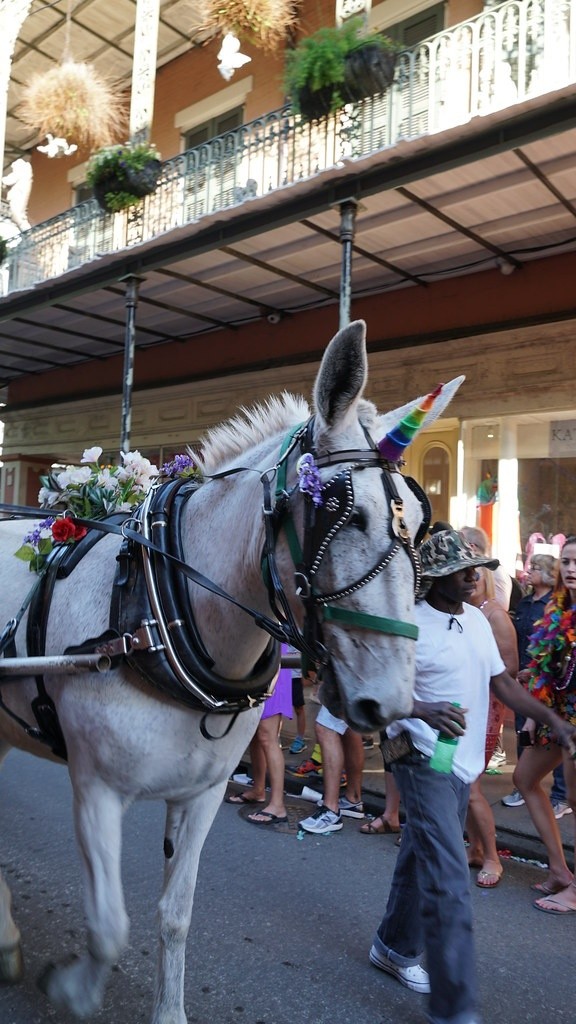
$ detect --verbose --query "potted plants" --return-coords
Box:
[280,15,408,128]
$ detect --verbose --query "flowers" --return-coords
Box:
[14,446,194,574]
[85,141,162,191]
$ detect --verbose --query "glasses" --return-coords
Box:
[531,565,545,574]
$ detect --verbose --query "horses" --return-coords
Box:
[1,320,466,1023]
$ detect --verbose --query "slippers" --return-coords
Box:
[531,880,559,894]
[533,883,576,914]
[476,866,500,887]
[224,792,264,804]
[358,815,400,834]
[248,811,287,824]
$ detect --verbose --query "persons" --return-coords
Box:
[225,519,576,1023]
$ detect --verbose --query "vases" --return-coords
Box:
[92,160,163,214]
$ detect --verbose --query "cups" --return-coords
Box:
[301,786,323,802]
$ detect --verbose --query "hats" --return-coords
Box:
[416,531,499,577]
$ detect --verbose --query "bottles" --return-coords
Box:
[485,769,504,775]
[430,703,460,774]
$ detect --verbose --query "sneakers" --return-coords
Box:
[336,767,347,786]
[299,805,344,833]
[368,945,432,993]
[317,795,365,818]
[503,787,528,806]
[290,736,307,753]
[283,757,322,777]
[487,750,507,768]
[551,798,572,819]
[360,737,375,749]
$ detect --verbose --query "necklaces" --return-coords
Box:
[522,585,576,748]
